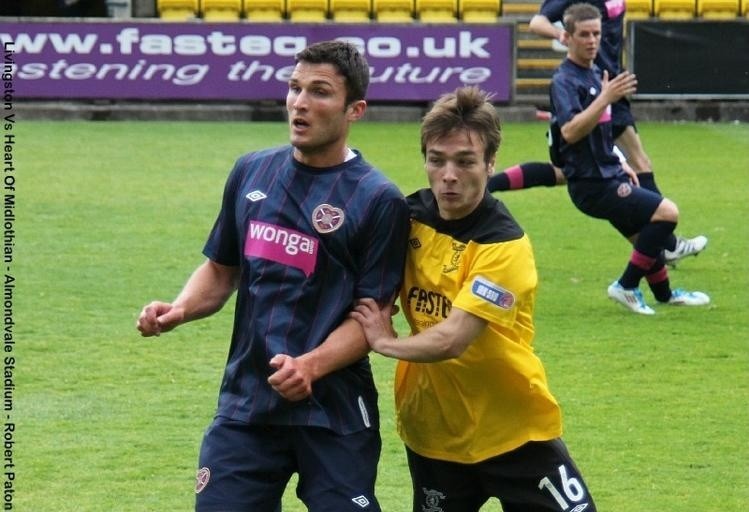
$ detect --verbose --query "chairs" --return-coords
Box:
[620,0,749,41]
[155,0,500,25]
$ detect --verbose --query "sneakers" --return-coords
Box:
[663,236,708,260]
[608,281,655,315]
[669,289,710,305]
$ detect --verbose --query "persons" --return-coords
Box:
[345,80,598,512]
[134,40,413,512]
[484,1,708,268]
[549,1,712,317]
